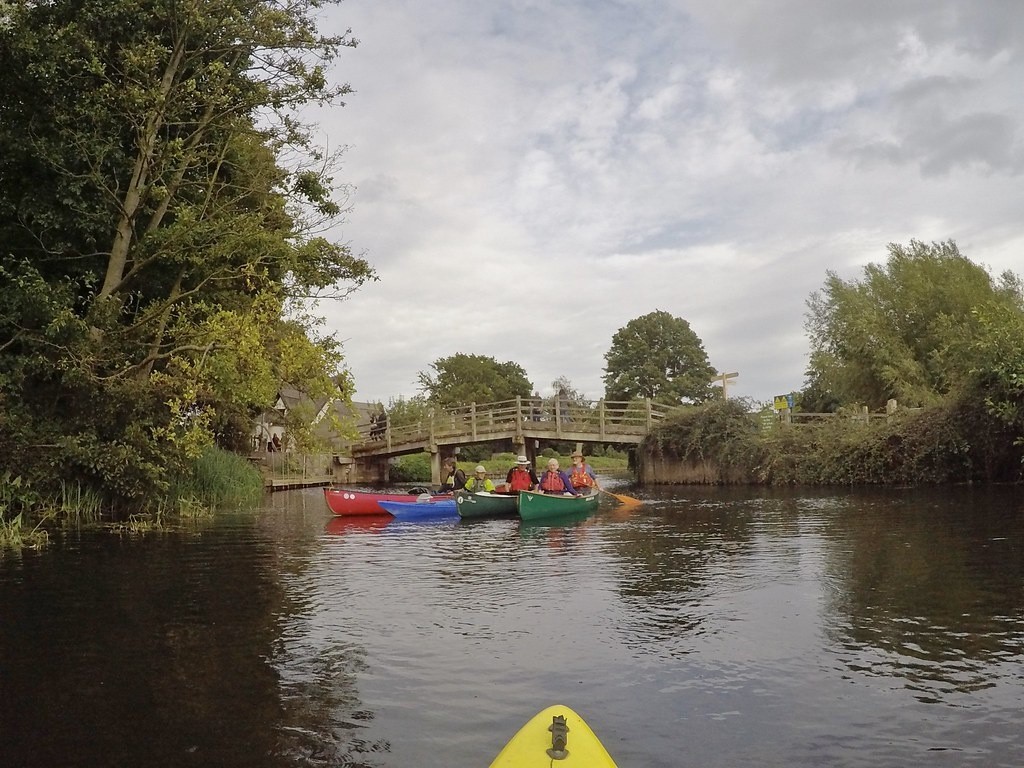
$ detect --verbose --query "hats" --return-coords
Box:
[570,451,585,463]
[475,466,487,473]
[514,456,531,465]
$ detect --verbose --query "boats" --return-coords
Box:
[515,483,602,521]
[322,481,455,517]
[450,486,537,520]
[377,492,459,518]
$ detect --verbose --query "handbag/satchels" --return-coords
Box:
[370,417,377,429]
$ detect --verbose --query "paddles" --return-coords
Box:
[571,480,638,503]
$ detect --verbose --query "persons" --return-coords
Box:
[437,458,468,496]
[273,433,298,451]
[564,451,601,495]
[538,457,583,497]
[464,466,496,493]
[369,411,387,442]
[528,389,570,421]
[506,456,540,496]
